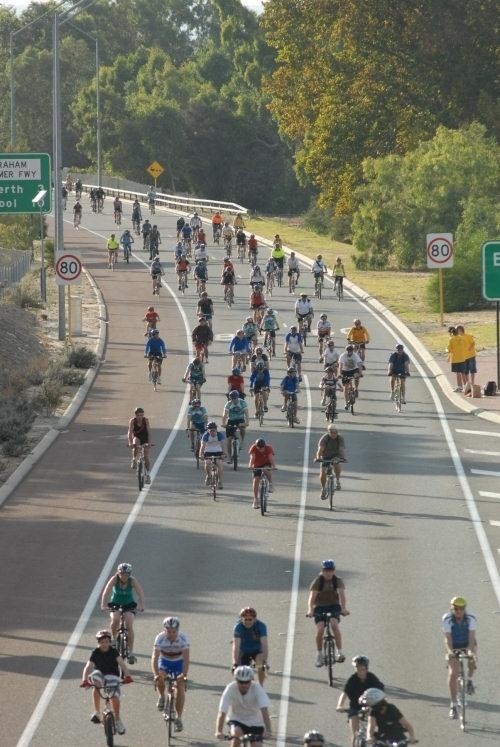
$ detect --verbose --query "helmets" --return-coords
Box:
[353,656,368,666]
[206,421,219,429]
[95,629,110,639]
[163,617,179,628]
[134,407,145,415]
[239,608,256,618]
[304,731,323,747]
[148,252,361,375]
[234,666,254,682]
[358,688,385,705]
[118,563,132,575]
[396,344,403,349]
[255,438,266,448]
[451,597,467,608]
[327,424,338,431]
[321,561,335,569]
[191,399,202,407]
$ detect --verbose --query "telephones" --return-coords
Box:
[54,249,83,287]
[426,233,454,270]
[0,153,51,215]
[480,239,500,301]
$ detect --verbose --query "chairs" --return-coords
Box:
[115,720,125,734]
[91,713,99,724]
[450,707,457,720]
[314,652,322,667]
[174,717,182,734]
[336,653,345,662]
[156,695,166,709]
[466,686,474,697]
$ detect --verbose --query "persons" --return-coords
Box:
[302,730,328,747]
[358,691,417,747]
[199,421,225,489]
[314,424,347,500]
[443,596,481,719]
[142,307,167,384]
[83,631,127,735]
[152,618,190,732]
[181,286,369,464]
[62,179,157,233]
[447,326,479,392]
[107,212,346,303]
[307,561,349,667]
[216,667,273,747]
[248,439,276,509]
[100,563,144,665]
[128,408,152,484]
[234,608,271,684]
[387,344,410,405]
[336,655,391,747]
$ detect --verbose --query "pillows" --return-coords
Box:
[451,597,467,608]
[95,629,110,639]
[321,561,335,569]
[134,407,145,415]
[255,438,266,448]
[327,424,338,431]
[118,563,132,575]
[358,688,385,705]
[234,666,254,682]
[206,421,219,429]
[163,617,179,628]
[304,731,323,747]
[353,656,368,666]
[239,608,256,618]
[191,399,202,407]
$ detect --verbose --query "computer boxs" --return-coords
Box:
[32,190,47,204]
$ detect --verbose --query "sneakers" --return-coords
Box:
[450,707,457,720]
[314,652,322,667]
[115,720,125,734]
[336,653,345,662]
[466,686,474,697]
[156,695,166,709]
[174,717,182,734]
[91,713,99,724]
